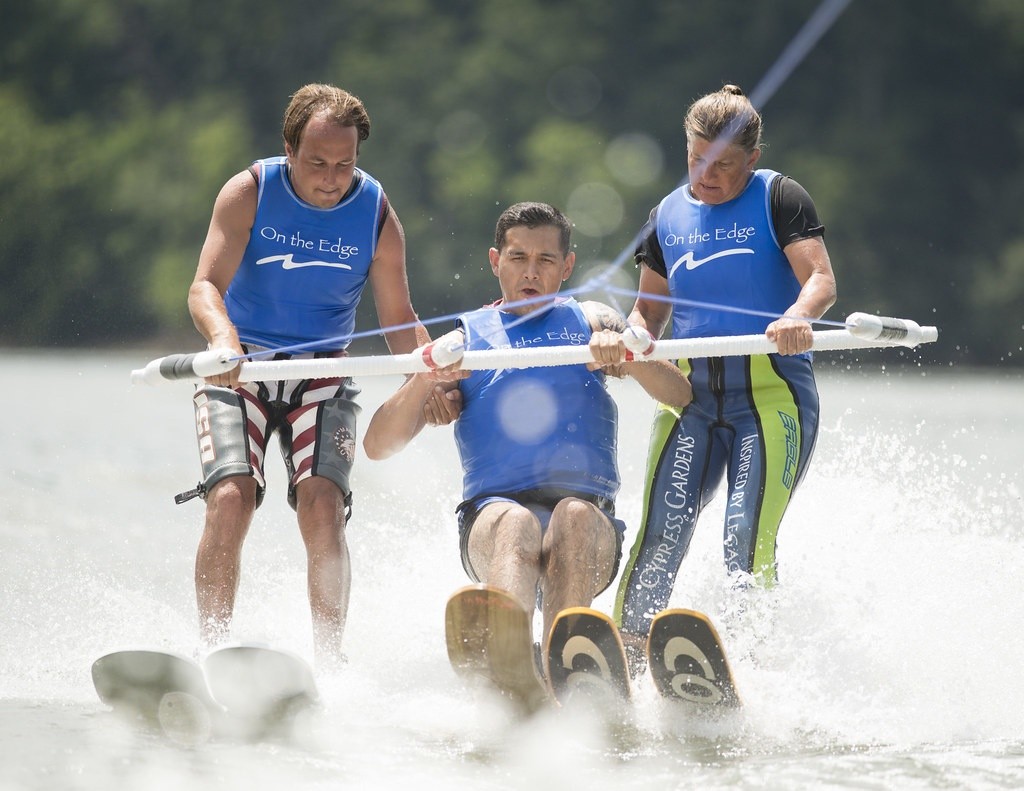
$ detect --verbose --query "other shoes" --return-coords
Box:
[626,643,647,681]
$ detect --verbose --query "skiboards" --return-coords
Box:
[87,585,739,747]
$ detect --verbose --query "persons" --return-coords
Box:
[614,79,821,679]
[174,77,464,659]
[363,197,694,680]
